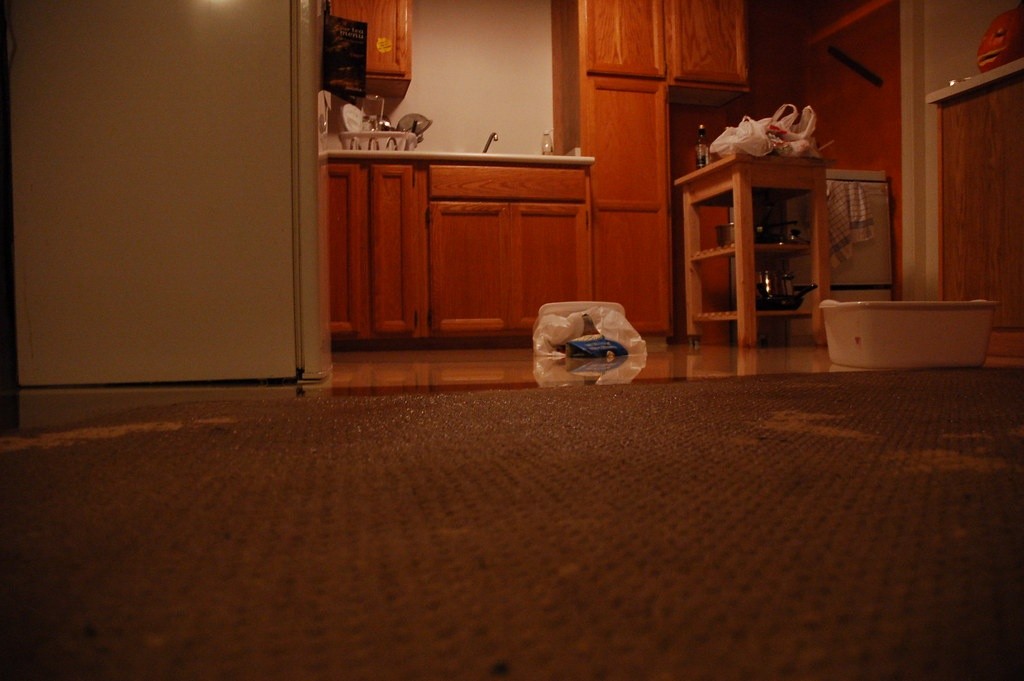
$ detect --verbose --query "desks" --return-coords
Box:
[674,153,835,350]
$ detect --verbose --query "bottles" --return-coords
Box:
[696,124,709,169]
[542,129,554,156]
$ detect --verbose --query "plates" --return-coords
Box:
[341,103,369,133]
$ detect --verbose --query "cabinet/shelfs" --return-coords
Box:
[326,0,416,98]
[553,0,753,342]
[325,163,594,344]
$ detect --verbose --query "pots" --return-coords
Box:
[732,272,817,309]
[715,219,799,248]
